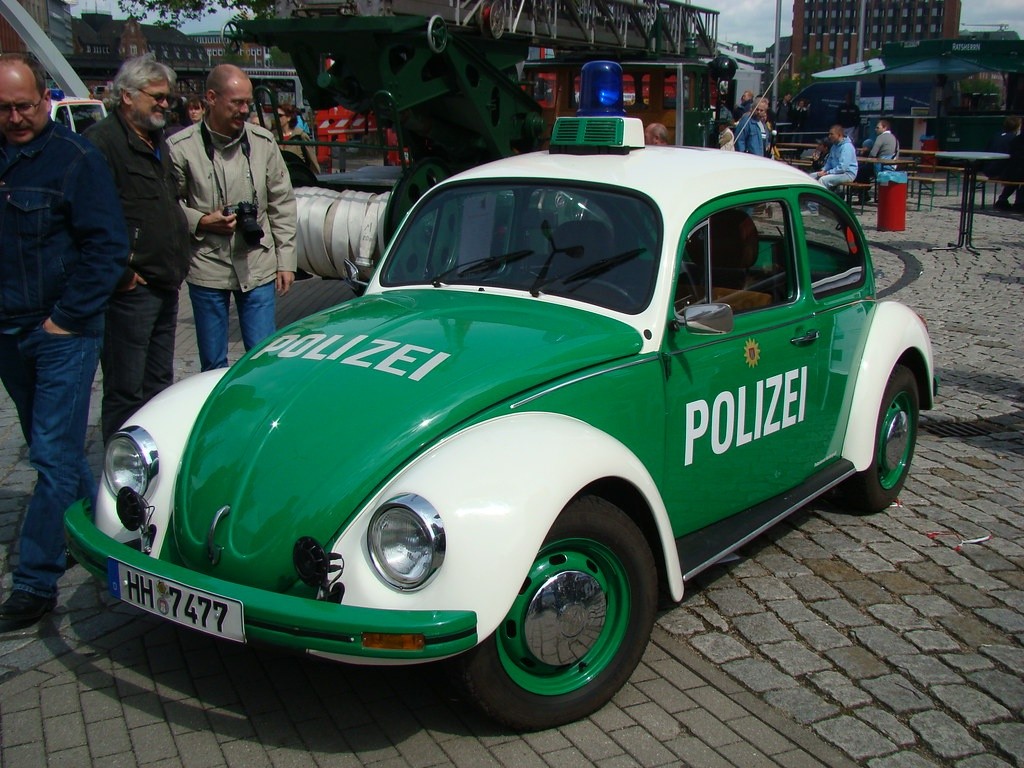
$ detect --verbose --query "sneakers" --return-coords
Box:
[65,548,78,571]
[0,590,58,632]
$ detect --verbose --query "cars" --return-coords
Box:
[56,60,937,730]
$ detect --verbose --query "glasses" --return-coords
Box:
[128,85,174,105]
[876,126,884,130]
[210,88,255,106]
[0,94,43,117]
[278,113,286,117]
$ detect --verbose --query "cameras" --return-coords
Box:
[222,201,264,245]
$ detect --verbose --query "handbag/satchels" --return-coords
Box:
[874,155,893,176]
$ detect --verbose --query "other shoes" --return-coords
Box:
[801,210,818,217]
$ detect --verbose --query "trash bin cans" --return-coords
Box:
[920,138,938,173]
[877,172,906,232]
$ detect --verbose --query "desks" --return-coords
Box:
[775,142,819,151]
[898,149,941,195]
[855,156,915,202]
[927,150,1010,252]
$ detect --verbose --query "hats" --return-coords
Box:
[862,139,873,149]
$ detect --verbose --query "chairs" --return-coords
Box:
[548,220,626,311]
[673,208,774,319]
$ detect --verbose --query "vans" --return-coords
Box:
[47,87,107,139]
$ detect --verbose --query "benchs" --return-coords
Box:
[775,146,1024,215]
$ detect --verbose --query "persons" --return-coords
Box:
[250,103,321,174]
[776,92,808,159]
[166,64,297,373]
[983,115,1024,213]
[164,112,185,140]
[718,90,774,215]
[187,94,206,124]
[82,53,192,448]
[801,124,858,217]
[836,91,861,129]
[857,120,899,204]
[644,123,669,145]
[957,93,976,112]
[0,53,131,634]
